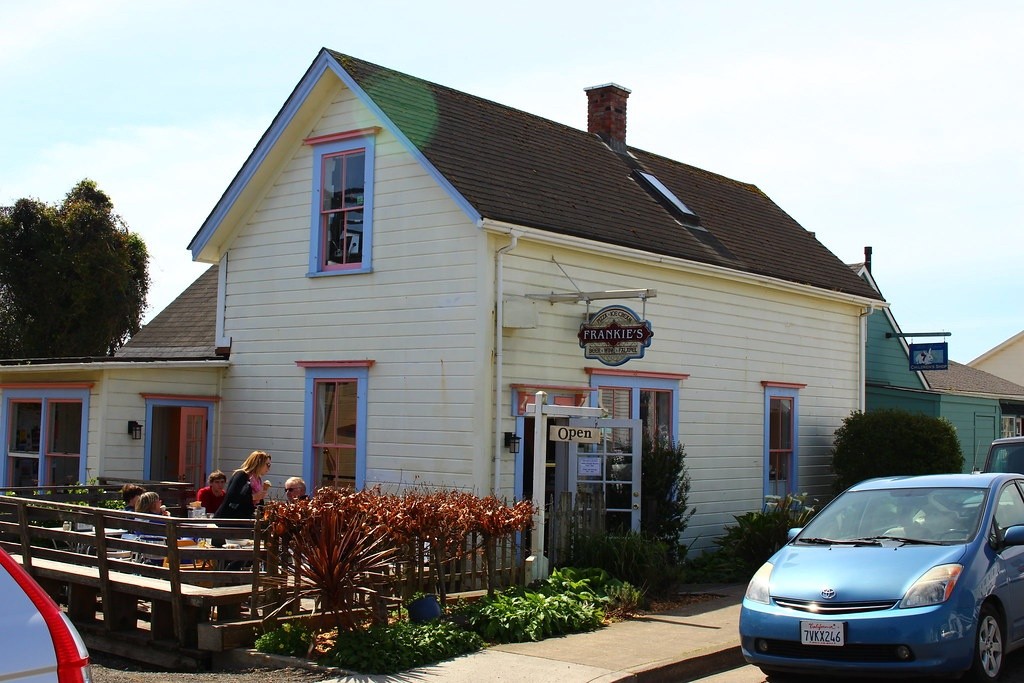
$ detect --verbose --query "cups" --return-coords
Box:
[198,537,205,547]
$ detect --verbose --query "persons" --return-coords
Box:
[121,484,145,520]
[134,492,170,562]
[197,470,227,513]
[285,477,308,505]
[213,451,271,528]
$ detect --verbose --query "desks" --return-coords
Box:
[47,527,128,567]
[175,544,289,570]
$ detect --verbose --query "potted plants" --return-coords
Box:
[402,592,441,623]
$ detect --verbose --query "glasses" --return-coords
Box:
[264,463,271,467]
[284,487,298,492]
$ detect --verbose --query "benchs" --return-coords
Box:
[0,483,438,647]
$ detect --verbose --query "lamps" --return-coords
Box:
[127,421,143,440]
[504,432,522,453]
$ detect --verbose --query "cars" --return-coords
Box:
[739,475,1023,683]
[983,436,1024,475]
[0,548,96,683]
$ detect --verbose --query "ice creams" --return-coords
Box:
[263,480,271,491]
[160,505,166,512]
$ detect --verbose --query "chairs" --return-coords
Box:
[163,540,217,619]
[135,535,167,564]
[84,538,133,568]
